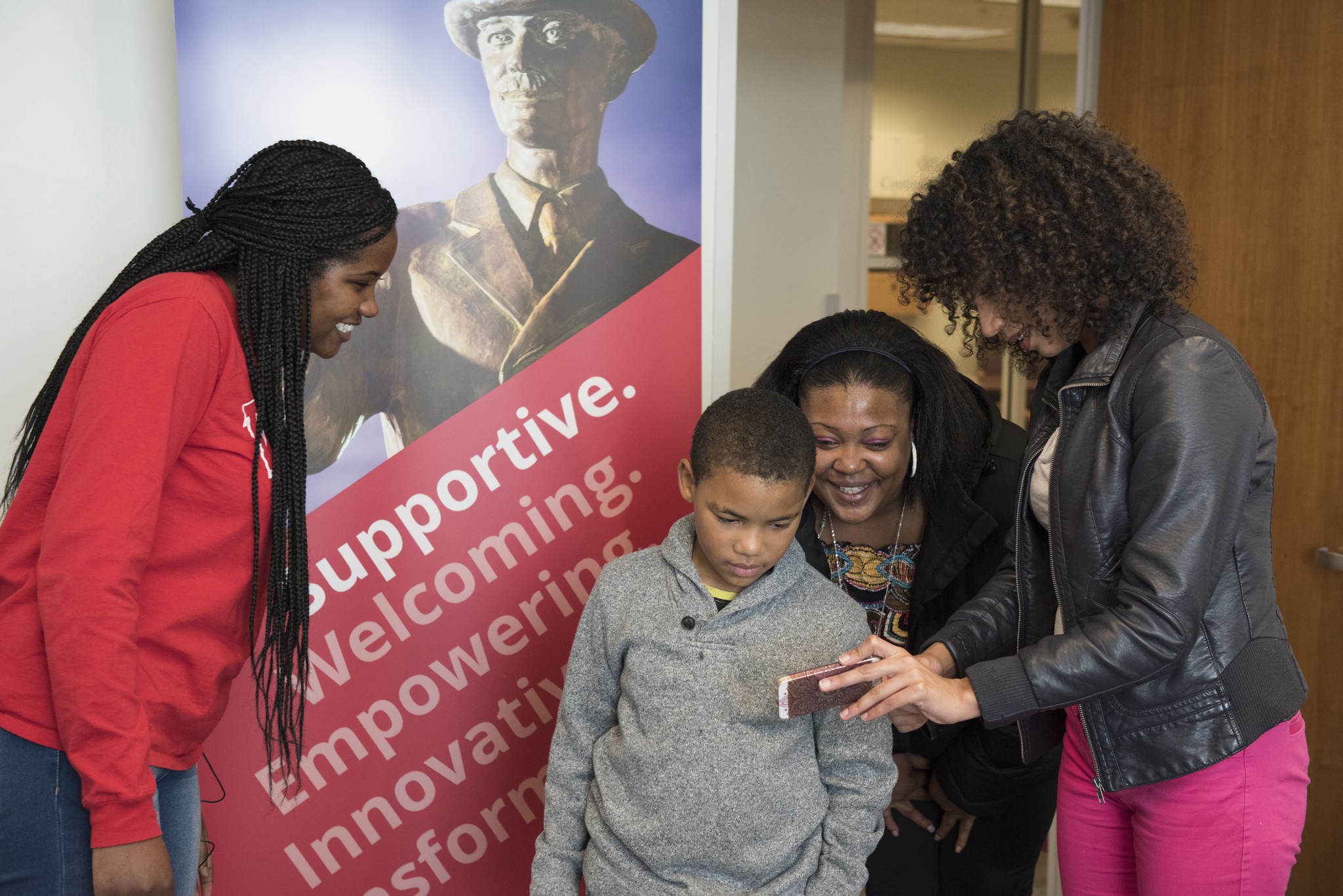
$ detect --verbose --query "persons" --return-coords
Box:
[0,140,399,896]
[751,309,1065,896]
[817,111,1309,893]
[530,386,896,896]
[304,0,696,473]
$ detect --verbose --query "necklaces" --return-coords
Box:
[820,508,910,638]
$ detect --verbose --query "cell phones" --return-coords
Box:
[774,652,883,720]
